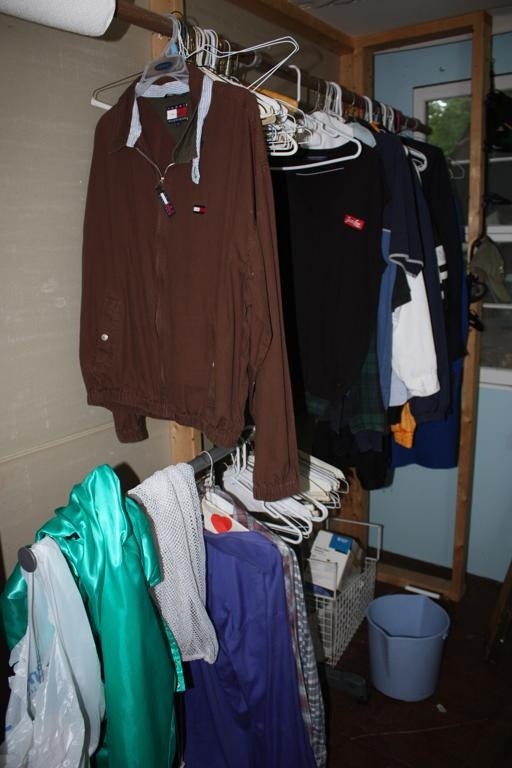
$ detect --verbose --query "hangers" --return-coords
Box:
[205,421,351,543]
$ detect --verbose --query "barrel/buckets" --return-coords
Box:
[367,593,451,703]
